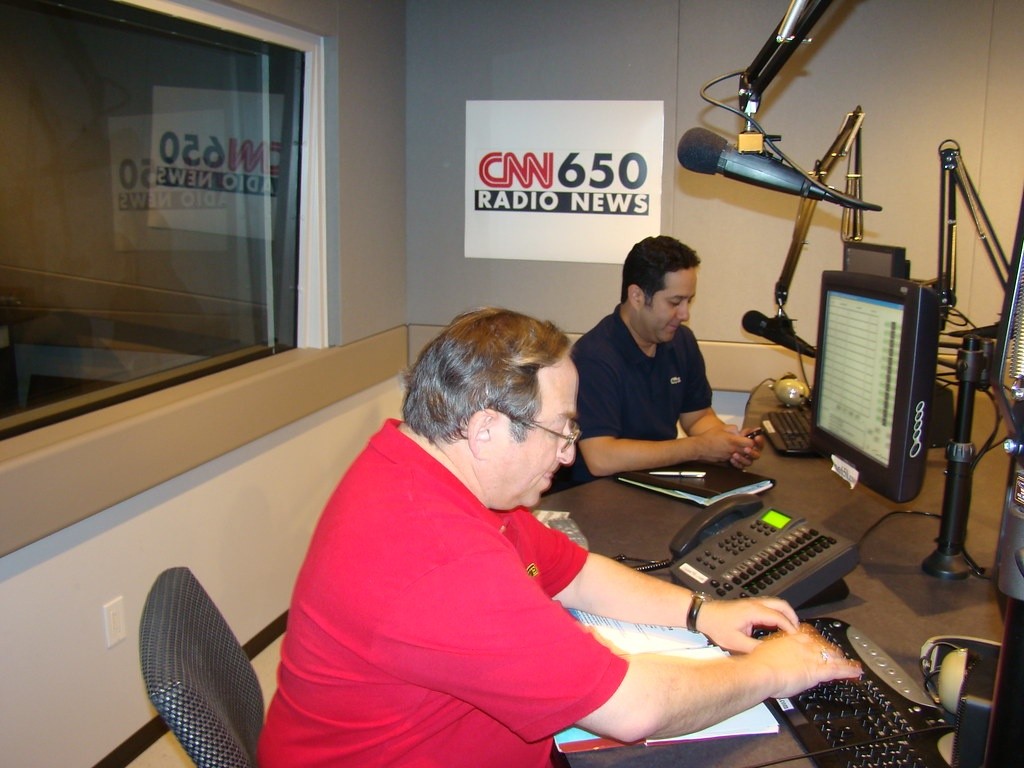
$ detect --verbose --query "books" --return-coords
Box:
[618,462,776,510]
[553,608,779,753]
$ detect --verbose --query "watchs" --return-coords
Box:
[687,591,711,633]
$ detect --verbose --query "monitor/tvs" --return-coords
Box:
[812,271,957,505]
[842,241,911,280]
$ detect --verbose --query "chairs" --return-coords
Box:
[137,567,264,768]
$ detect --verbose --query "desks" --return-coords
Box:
[524,376,1024,768]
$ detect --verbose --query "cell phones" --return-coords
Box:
[745,429,763,439]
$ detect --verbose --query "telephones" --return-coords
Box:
[668,494,863,611]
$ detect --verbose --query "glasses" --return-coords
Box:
[525,420,583,453]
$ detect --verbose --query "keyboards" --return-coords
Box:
[760,409,820,458]
[749,617,955,768]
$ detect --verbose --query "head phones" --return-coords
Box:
[919,635,1004,715]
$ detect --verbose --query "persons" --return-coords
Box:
[543,235,764,498]
[257,306,863,768]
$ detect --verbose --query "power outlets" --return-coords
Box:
[102,595,129,648]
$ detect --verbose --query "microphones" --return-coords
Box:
[741,310,816,359]
[677,127,868,210]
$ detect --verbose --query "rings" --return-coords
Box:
[820,649,827,662]
